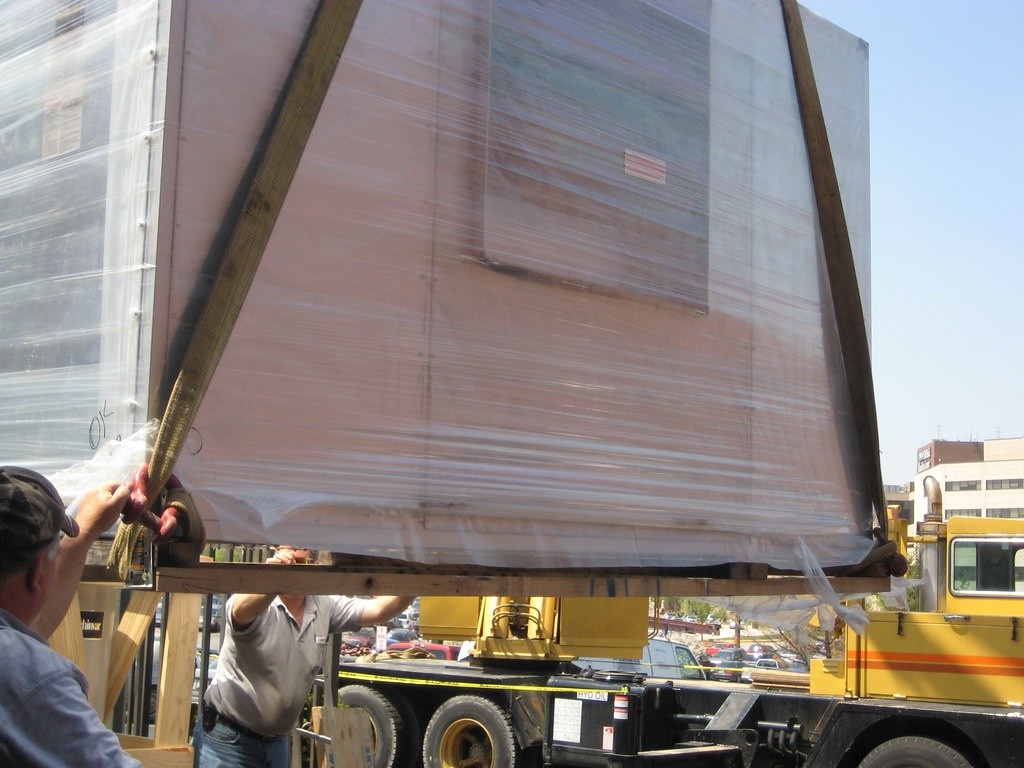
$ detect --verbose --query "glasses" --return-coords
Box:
[294,557,315,564]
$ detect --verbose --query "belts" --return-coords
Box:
[215,714,283,743]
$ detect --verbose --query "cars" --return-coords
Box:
[699,642,828,684]
[145,590,222,735]
[339,597,463,661]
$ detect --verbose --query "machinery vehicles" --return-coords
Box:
[305,474,1023,768]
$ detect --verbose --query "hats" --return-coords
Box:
[0,465,79,551]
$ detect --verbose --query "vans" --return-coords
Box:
[570,635,708,681]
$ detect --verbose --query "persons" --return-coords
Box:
[192,545,416,768]
[0,465,144,768]
